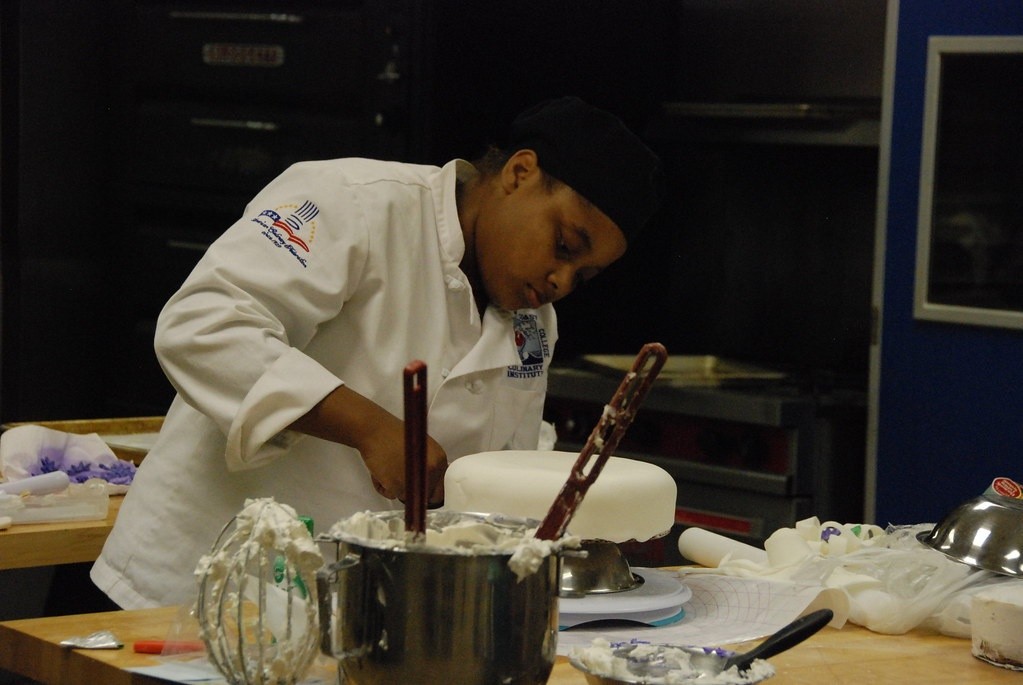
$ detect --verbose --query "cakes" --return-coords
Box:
[444,450,676,543]
[971,586,1023,666]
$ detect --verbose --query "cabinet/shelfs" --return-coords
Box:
[97,0,437,417]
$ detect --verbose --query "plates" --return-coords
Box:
[580,353,786,386]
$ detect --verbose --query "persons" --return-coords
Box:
[78,95,668,615]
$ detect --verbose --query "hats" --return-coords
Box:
[493,98,662,257]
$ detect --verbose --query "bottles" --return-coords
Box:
[291,517,331,656]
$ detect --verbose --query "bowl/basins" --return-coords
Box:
[568,639,764,685]
[916,494,1023,578]
[969,581,1023,671]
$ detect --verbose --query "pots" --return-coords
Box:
[315,510,587,685]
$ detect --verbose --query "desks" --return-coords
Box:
[0,564,1023,685]
[0,414,166,685]
